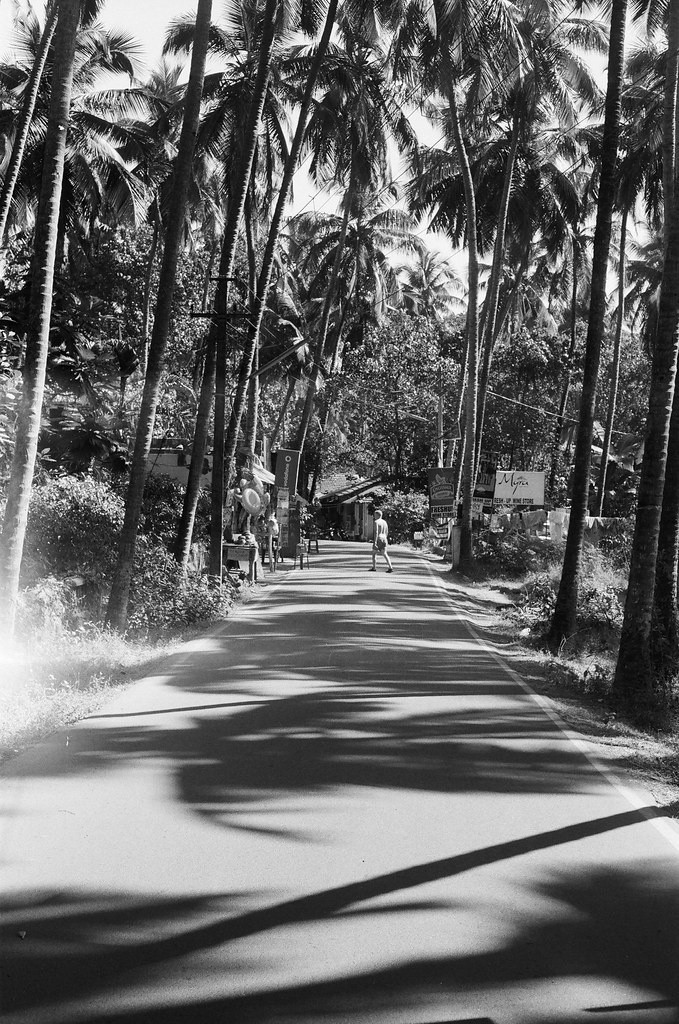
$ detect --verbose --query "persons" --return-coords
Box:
[369,510,394,573]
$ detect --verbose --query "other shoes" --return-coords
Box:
[386,568,393,573]
[368,567,376,571]
